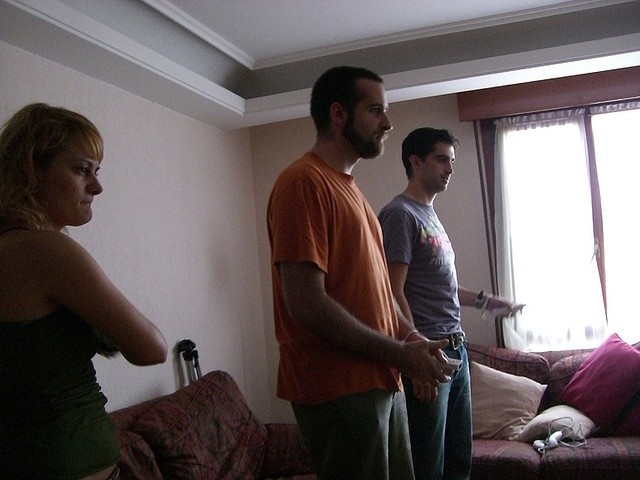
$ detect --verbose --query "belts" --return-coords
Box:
[419,331,467,351]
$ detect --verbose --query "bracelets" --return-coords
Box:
[474,290,485,310]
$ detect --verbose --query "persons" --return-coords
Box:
[377,131,516,479]
[266,66,459,479]
[0,103,168,478]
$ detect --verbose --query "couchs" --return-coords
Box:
[465,333,640,480]
[104,369,322,480]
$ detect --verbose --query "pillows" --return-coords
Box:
[470,359,547,441]
[611,404,639,437]
[550,354,591,399]
[555,332,639,433]
[505,403,598,441]
[467,341,550,385]
[133,370,269,480]
